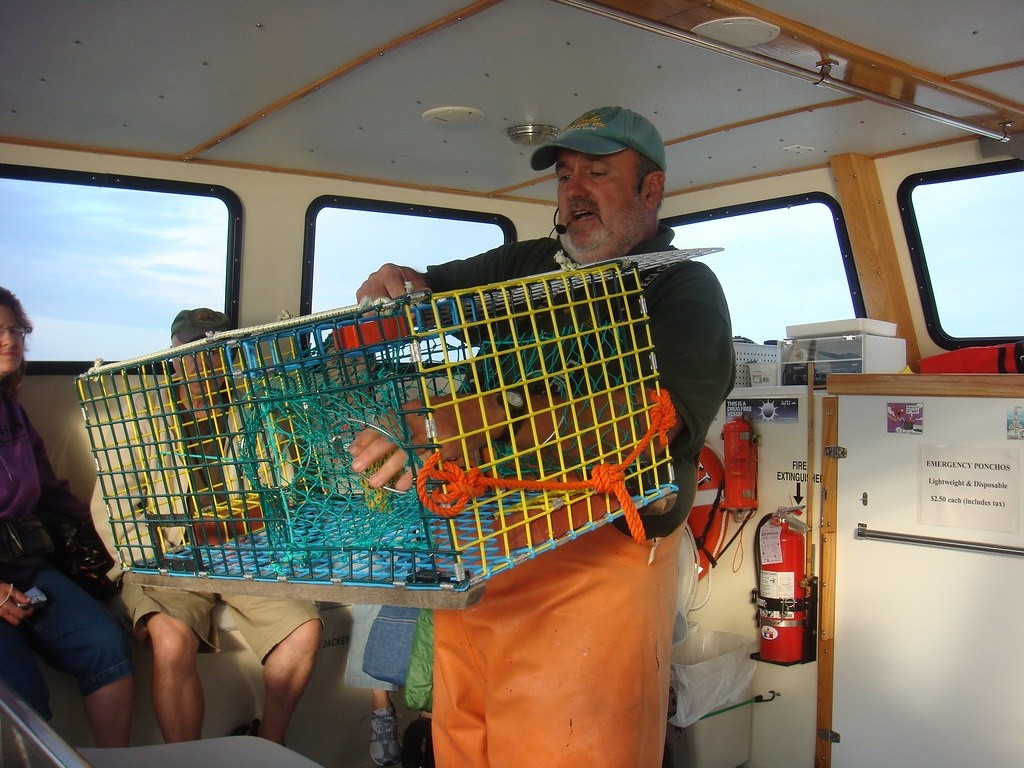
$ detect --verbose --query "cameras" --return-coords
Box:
[13,586,48,609]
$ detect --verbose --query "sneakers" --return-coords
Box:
[359,701,403,766]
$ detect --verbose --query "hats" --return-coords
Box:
[530,106,666,173]
[171,309,232,344]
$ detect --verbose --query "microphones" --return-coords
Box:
[553,207,567,234]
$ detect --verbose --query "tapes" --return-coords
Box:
[330,314,409,350]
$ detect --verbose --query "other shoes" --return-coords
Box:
[232,719,262,738]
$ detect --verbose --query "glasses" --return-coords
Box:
[0,326,28,341]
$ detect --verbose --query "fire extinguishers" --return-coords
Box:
[720,416,760,511]
[755,506,807,661]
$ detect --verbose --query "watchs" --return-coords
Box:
[497,390,526,442]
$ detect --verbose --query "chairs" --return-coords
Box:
[0,679,325,768]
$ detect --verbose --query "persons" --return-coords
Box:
[0,288,136,748]
[349,106,737,768]
[90,307,325,747]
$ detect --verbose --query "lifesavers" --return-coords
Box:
[684,442,731,583]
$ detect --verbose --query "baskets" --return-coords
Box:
[733,342,781,388]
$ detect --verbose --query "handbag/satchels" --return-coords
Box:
[0,513,55,570]
[42,512,121,600]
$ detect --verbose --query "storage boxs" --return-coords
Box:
[780,335,906,389]
[731,338,778,388]
